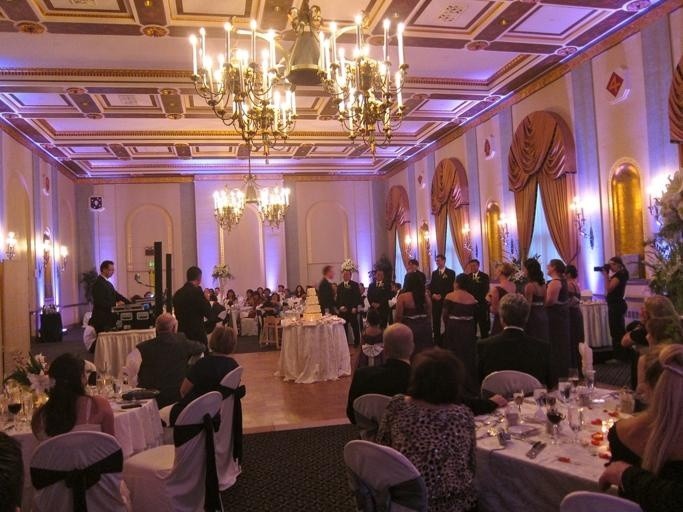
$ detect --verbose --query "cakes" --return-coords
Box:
[302,287,322,322]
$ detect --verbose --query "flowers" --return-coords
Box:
[340,258,357,272]
[212,264,234,279]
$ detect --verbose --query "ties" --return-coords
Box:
[473,274,476,279]
[439,270,442,276]
[377,283,380,287]
[344,284,348,288]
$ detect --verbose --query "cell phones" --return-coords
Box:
[527,441,546,459]
[121,404,141,409]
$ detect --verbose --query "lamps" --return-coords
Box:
[44,238,53,269]
[404,234,415,260]
[6,230,16,260]
[422,230,433,256]
[60,245,71,273]
[496,218,509,249]
[185,0,410,166]
[462,224,474,256]
[212,139,288,234]
[570,195,591,240]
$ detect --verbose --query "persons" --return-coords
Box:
[0,260,240,511]
[602,257,628,344]
[246,284,310,322]
[316,254,578,390]
[597,295,683,512]
[346,323,477,510]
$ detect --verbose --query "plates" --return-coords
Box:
[333,317,340,322]
[128,386,160,398]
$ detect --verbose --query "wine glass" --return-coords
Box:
[87,366,129,408]
[497,368,638,455]
[324,308,332,319]
[2,374,51,428]
[223,295,303,325]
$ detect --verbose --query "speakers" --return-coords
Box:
[89,195,103,211]
[39,304,63,343]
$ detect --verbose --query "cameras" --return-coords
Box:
[497,431,511,445]
[594,264,610,274]
